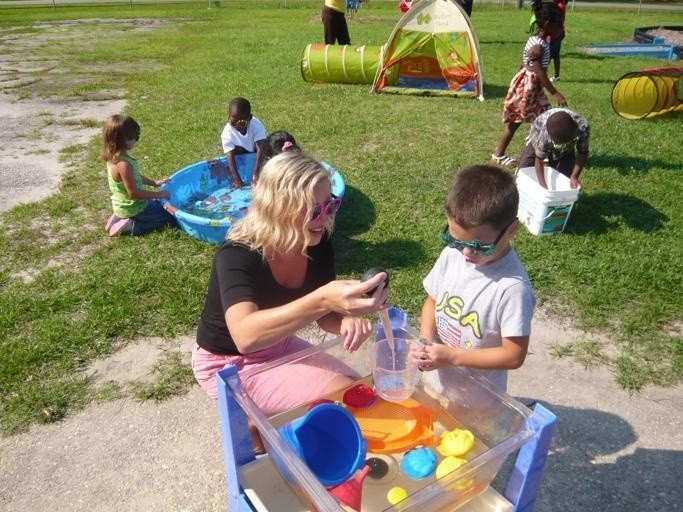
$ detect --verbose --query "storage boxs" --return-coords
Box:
[225,317,547,512]
[238,455,515,511]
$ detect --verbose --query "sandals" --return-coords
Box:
[549,76,561,83]
[490,154,518,167]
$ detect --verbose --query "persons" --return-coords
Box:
[263,132,299,159]
[525,0,569,82]
[409,165,534,394]
[221,97,270,189]
[322,0,352,46]
[99,114,171,234]
[492,9,569,169]
[517,109,591,196]
[191,151,390,455]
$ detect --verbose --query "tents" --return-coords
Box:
[370,0,487,103]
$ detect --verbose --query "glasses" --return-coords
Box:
[228,120,248,128]
[302,193,341,221]
[105,212,119,230]
[132,135,141,143]
[441,217,519,256]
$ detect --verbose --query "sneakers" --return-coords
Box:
[106,219,136,238]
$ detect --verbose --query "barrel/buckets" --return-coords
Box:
[270,402,363,487]
